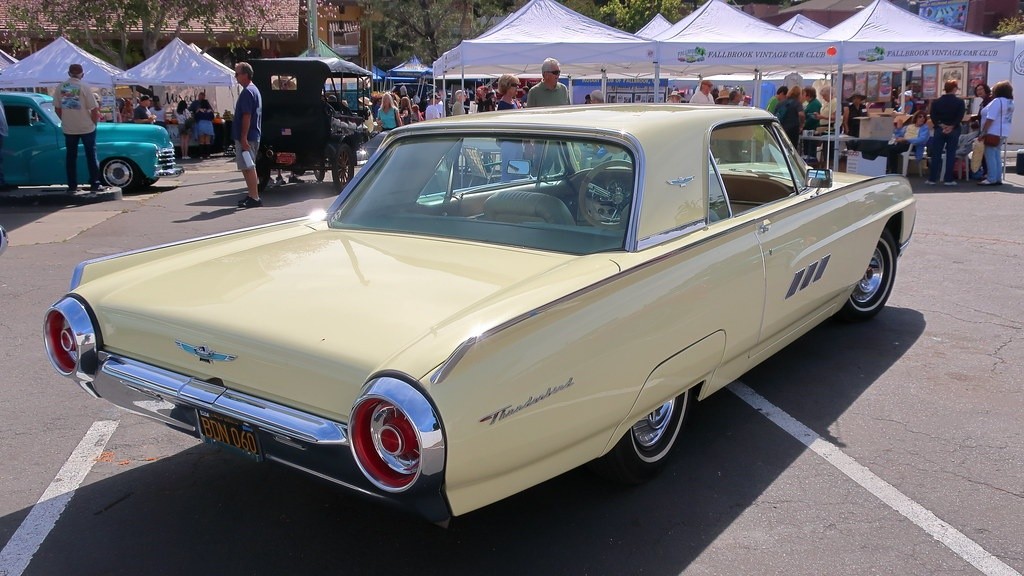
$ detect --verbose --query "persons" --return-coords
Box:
[190,92,215,158]
[54,64,110,192]
[329,57,604,182]
[91,92,167,129]
[665,80,1015,185]
[0,73,18,191]
[232,61,263,208]
[176,92,193,159]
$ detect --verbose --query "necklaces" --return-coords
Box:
[504,97,513,103]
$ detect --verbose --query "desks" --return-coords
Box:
[463,143,501,152]
[799,134,857,168]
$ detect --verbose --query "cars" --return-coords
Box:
[43,98,920,528]
[0,91,185,191]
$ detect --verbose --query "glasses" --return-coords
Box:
[547,70,560,75]
[511,83,521,87]
[235,72,239,77]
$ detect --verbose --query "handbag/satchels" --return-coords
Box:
[984,134,1000,146]
[185,119,192,128]
[523,141,534,160]
[971,138,984,173]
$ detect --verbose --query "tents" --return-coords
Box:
[271,0,656,118]
[634,0,1016,182]
[0,36,244,122]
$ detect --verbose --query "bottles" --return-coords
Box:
[242,150,254,168]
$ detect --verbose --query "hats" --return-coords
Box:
[430,95,441,99]
[69,64,83,74]
[140,95,149,101]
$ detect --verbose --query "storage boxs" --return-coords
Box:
[853,113,894,140]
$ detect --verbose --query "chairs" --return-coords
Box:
[900,143,923,177]
[464,146,502,187]
[940,131,979,182]
[483,191,576,226]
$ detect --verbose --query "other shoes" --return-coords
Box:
[977,175,1003,185]
[182,156,191,160]
[199,156,210,159]
[67,187,78,193]
[90,182,109,192]
[802,158,818,164]
[924,180,936,184]
[944,181,958,185]
[272,177,286,186]
[238,196,263,208]
[289,176,305,183]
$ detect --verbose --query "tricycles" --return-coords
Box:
[245,55,375,191]
